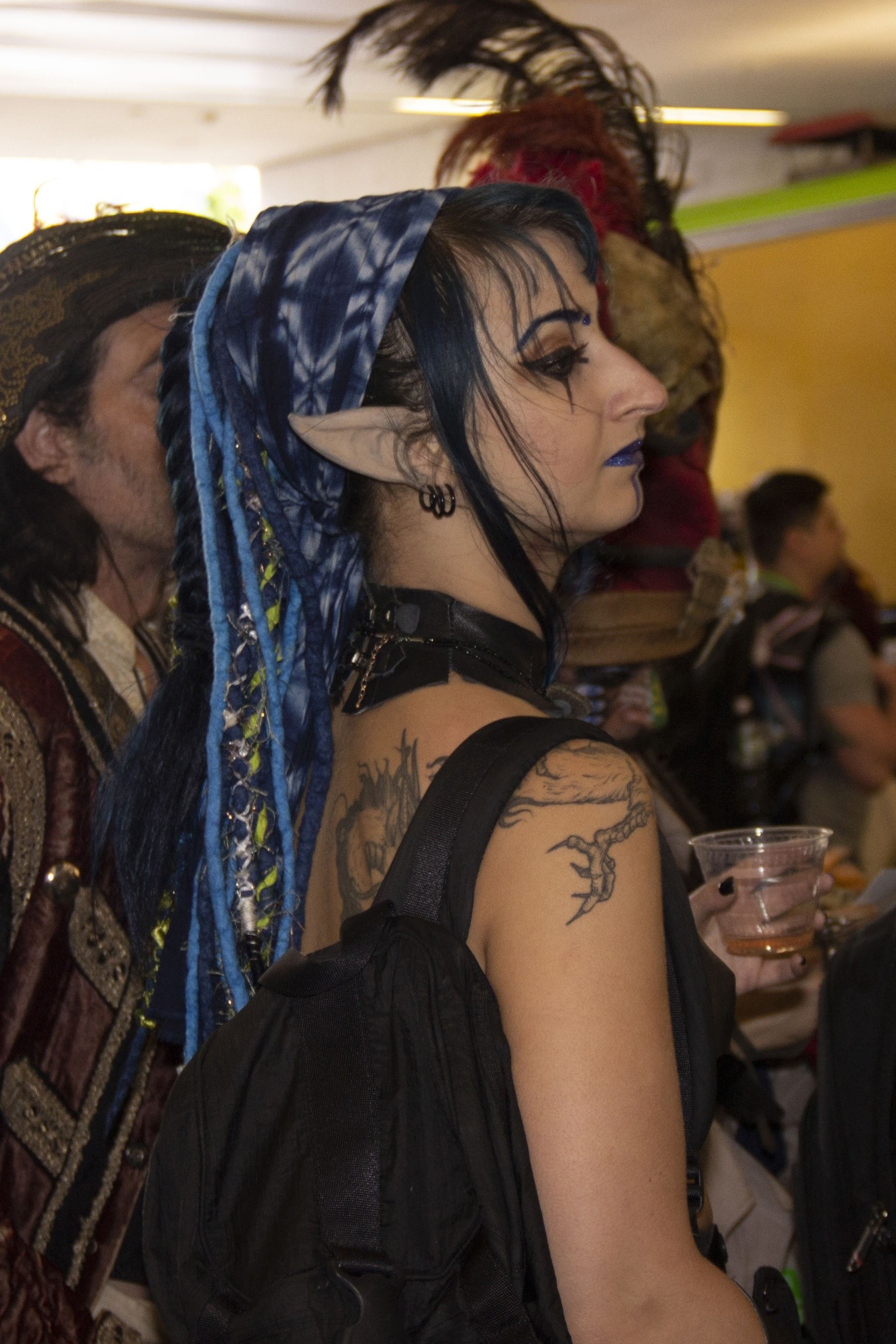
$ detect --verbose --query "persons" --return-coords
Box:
[0,0,896,1344]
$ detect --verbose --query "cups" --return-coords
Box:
[686,824,834,956]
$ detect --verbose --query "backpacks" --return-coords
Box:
[796,912,895,1344]
[665,594,839,824]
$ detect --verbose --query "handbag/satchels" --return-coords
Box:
[135,717,730,1344]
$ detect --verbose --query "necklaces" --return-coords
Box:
[355,633,552,708]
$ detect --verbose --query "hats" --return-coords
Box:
[0,211,231,446]
